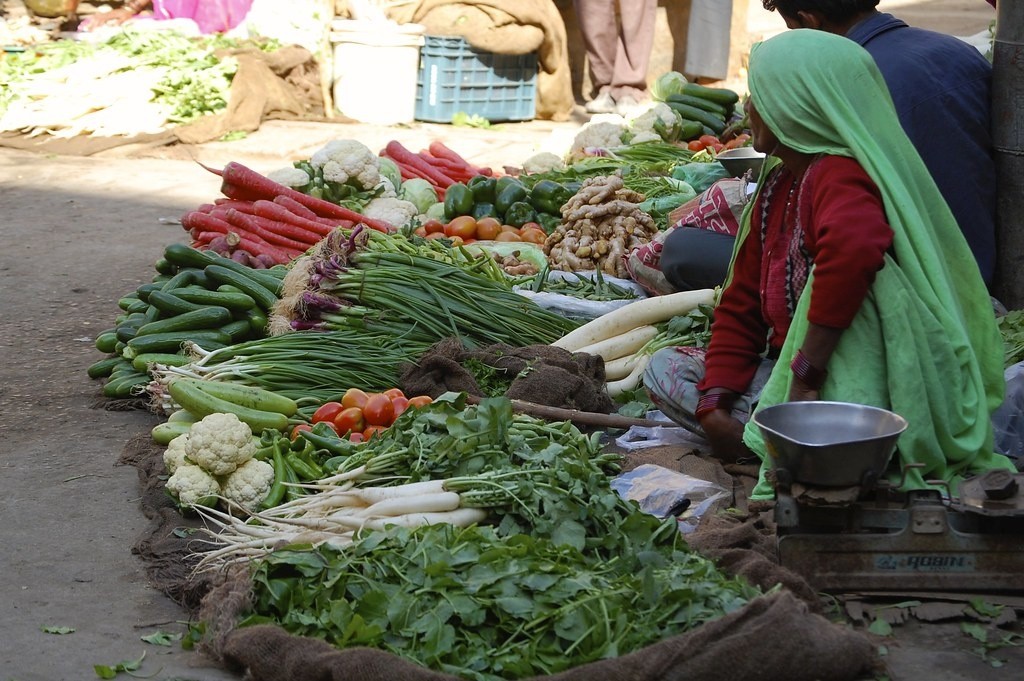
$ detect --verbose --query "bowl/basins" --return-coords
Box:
[714,147,766,183]
[753,401,908,489]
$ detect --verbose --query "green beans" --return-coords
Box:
[508,415,623,476]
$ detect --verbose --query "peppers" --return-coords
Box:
[444,174,581,234]
[253,423,359,513]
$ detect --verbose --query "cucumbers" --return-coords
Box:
[86,243,290,398]
[665,83,739,138]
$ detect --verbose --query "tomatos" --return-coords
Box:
[414,216,548,244]
[291,388,433,442]
[688,134,750,153]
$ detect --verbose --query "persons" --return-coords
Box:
[662,0,995,291]
[89,0,254,34]
[644,28,1010,490]
[572,0,735,114]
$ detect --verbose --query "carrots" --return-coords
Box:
[379,139,511,202]
[182,158,397,264]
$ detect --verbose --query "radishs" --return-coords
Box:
[551,288,715,396]
[183,466,484,582]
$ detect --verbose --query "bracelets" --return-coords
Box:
[695,393,734,415]
[121,0,143,15]
[790,351,828,389]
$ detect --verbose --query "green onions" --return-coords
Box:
[147,226,582,417]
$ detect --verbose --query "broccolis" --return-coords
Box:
[267,138,428,230]
[522,101,677,163]
[163,413,274,520]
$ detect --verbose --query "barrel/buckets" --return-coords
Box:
[330,20,425,124]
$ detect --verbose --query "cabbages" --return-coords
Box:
[650,72,687,102]
[378,157,449,225]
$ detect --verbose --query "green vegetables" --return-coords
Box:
[519,157,682,199]
[0,29,282,125]
[997,309,1024,370]
[94,494,763,681]
